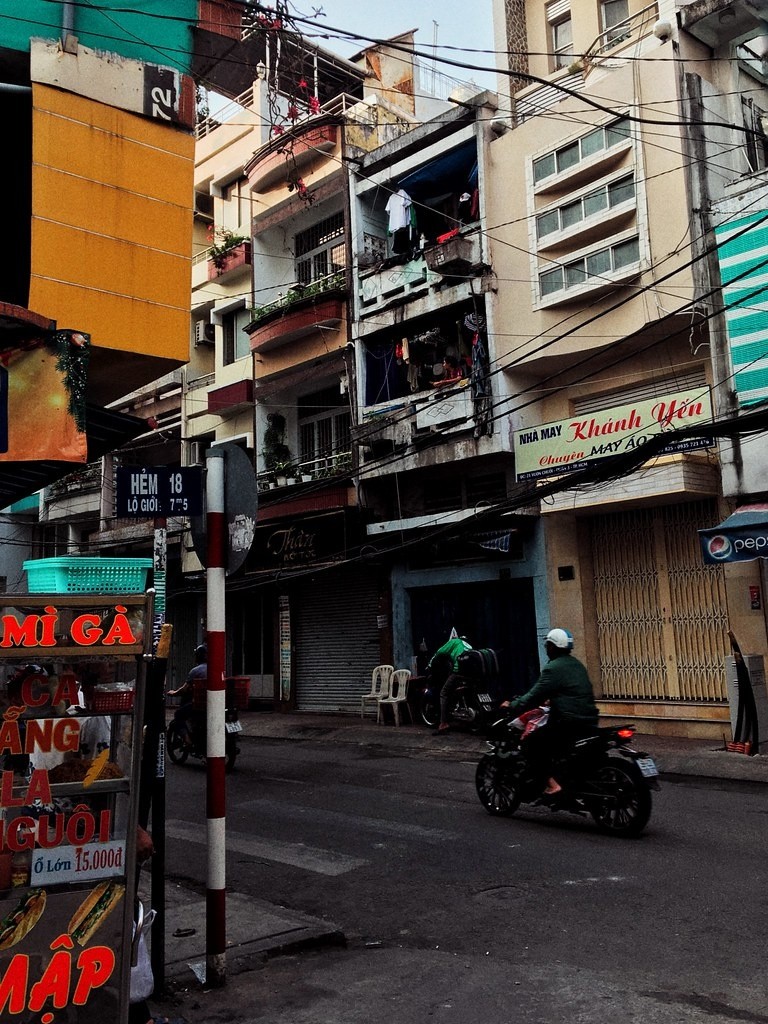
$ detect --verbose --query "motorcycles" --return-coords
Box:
[413,661,497,738]
[475,694,665,839]
[166,688,244,776]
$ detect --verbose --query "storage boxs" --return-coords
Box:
[193,676,250,709]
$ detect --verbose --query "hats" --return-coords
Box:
[194,645,206,657]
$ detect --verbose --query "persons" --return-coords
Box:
[422,635,475,736]
[497,627,600,800]
[166,643,208,755]
[432,356,464,388]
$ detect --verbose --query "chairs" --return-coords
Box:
[360,664,395,725]
[376,669,414,727]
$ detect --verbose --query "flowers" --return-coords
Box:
[204,224,251,255]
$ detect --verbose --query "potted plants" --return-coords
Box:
[52,465,100,495]
[268,460,312,489]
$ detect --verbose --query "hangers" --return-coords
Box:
[392,184,399,194]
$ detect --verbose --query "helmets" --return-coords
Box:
[545,628,574,649]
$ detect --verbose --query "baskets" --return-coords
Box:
[23,556,153,594]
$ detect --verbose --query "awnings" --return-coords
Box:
[697,498,768,567]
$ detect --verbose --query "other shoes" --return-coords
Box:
[432,726,450,735]
[179,744,194,753]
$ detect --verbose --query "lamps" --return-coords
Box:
[652,18,673,40]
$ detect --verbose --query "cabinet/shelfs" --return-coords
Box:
[0,588,156,1024]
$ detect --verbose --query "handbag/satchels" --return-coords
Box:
[458,647,498,673]
[506,706,551,740]
[130,908,157,1002]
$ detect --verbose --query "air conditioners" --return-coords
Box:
[195,319,216,345]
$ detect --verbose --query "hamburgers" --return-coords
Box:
[68,881,125,946]
[0,889,46,950]
